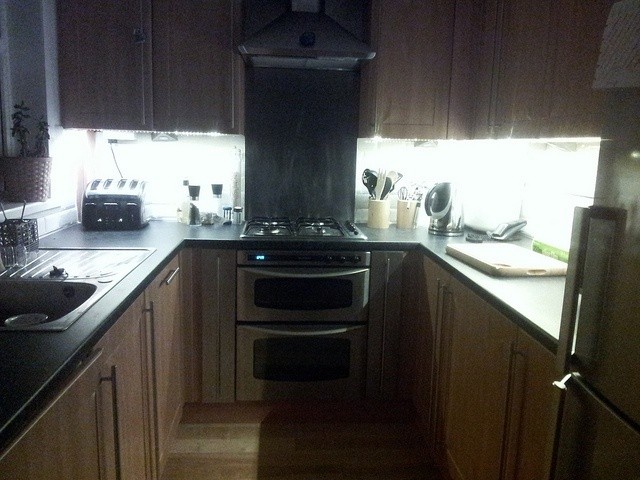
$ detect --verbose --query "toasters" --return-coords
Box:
[82,178,153,227]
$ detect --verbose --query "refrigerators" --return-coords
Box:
[549,93,640,480]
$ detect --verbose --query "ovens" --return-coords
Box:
[236,251,367,408]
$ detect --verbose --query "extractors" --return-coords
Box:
[238,1,375,73]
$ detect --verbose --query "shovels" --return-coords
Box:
[380,177,393,200]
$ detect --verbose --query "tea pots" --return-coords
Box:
[426,182,464,237]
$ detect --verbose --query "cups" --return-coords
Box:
[395,199,420,228]
[369,200,392,227]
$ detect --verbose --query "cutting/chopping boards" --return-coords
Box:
[445,240,568,278]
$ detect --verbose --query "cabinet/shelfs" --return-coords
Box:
[145,254,185,478]
[2,291,152,480]
[402,244,516,480]
[500,323,565,478]
[56,0,233,133]
[475,0,615,137]
[366,1,477,137]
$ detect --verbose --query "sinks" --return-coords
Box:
[0,280,99,330]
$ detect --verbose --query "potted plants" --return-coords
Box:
[0,101,51,202]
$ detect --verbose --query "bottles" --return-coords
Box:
[222,207,231,223]
[189,185,200,223]
[233,207,243,224]
[210,183,223,223]
[178,180,189,221]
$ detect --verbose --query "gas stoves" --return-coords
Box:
[241,217,367,243]
